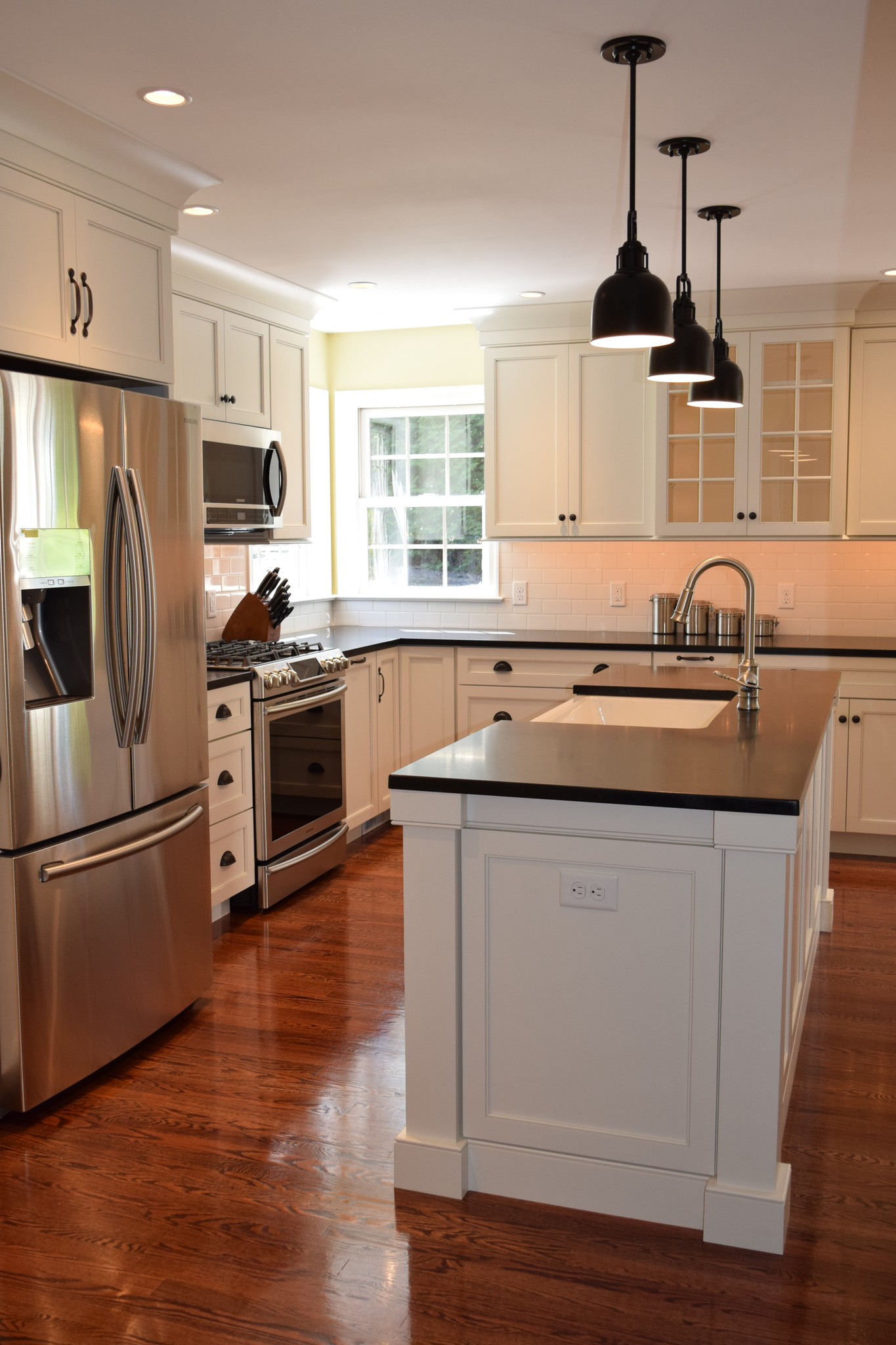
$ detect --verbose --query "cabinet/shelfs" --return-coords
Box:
[172,293,271,428]
[208,680,255,908]
[452,645,652,741]
[0,163,175,385]
[742,654,895,837]
[485,326,896,537]
[342,646,400,831]
[270,699,340,800]
[399,645,456,768]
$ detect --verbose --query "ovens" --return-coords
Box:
[248,675,353,911]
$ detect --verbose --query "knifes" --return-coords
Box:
[255,565,296,631]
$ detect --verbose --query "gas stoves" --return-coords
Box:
[207,636,351,700]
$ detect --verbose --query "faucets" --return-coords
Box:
[669,556,765,710]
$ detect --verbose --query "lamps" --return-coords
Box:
[647,137,716,385]
[686,206,745,409]
[588,34,675,351]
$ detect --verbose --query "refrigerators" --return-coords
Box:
[2,355,216,1124]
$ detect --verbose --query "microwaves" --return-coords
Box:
[202,418,287,543]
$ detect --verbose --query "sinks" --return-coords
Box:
[524,685,740,729]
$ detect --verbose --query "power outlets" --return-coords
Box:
[778,582,795,610]
[560,870,619,912]
[512,580,528,606]
[610,581,627,607]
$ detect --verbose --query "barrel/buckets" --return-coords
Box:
[713,607,745,635]
[683,599,712,635]
[650,592,679,636]
[747,614,778,636]
